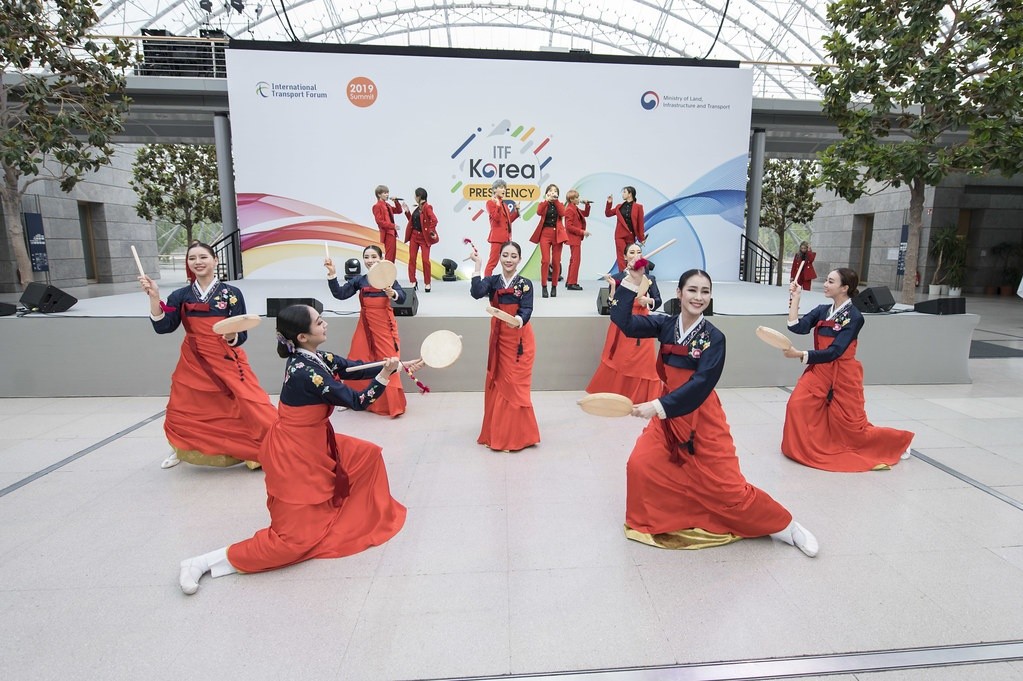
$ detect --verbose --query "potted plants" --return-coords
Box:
[986,240,1022,298]
[927,221,965,297]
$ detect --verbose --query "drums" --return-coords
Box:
[420,330,462,369]
[367,260,397,289]
[486,306,520,326]
[581,392,633,417]
[755,326,793,350]
[212,314,262,334]
[635,275,649,299]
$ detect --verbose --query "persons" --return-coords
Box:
[372,185,403,263]
[483,179,520,276]
[323,245,406,419]
[178,305,424,594]
[790,241,817,291]
[585,243,662,404]
[402,188,439,292]
[565,190,591,290]
[609,255,821,558]
[470,242,541,451]
[529,184,570,297]
[138,242,278,470]
[605,187,646,272]
[782,268,915,472]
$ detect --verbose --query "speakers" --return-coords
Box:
[852,286,895,314]
[267,298,323,318]
[389,287,419,317]
[597,287,611,315]
[20,281,78,313]
[0,302,17,316]
[663,297,713,316]
[913,297,967,315]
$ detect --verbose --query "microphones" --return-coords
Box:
[389,197,404,200]
[580,200,594,203]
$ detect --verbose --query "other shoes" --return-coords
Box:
[551,286,556,297]
[410,282,418,291]
[424,284,431,292]
[179,559,199,595]
[900,435,915,460]
[161,453,181,469]
[542,288,548,298]
[245,460,261,469]
[565,282,582,290]
[793,521,818,556]
